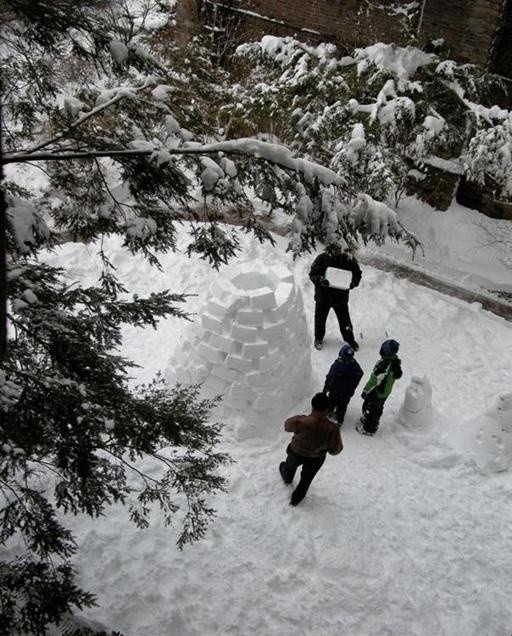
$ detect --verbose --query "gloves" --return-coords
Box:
[319,275,330,287]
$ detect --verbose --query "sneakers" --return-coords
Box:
[278,461,293,483]
[354,424,374,436]
[314,340,323,349]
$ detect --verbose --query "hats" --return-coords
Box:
[381,338,400,356]
[338,344,355,359]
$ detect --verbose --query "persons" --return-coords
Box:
[278,392,343,505]
[307,241,360,352]
[323,345,364,427]
[355,339,403,435]
